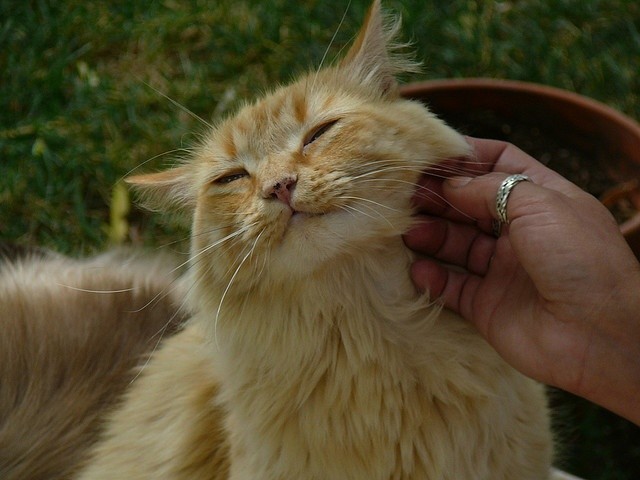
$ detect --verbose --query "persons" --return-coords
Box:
[402,135,639,423]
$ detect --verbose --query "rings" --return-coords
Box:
[495,173,534,225]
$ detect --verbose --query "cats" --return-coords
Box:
[1,0,570,479]
